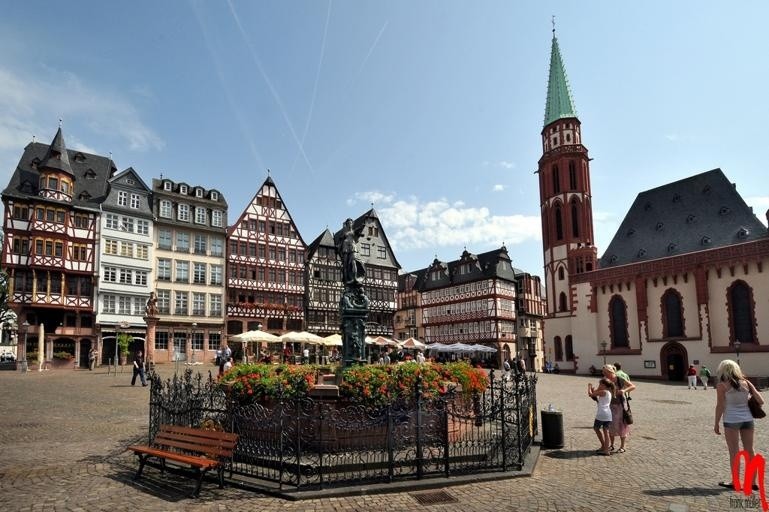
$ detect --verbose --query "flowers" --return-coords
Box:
[216,357,492,402]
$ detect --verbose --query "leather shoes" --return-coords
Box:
[751,484,758,490]
[719,481,733,489]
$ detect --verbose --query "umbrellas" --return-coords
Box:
[226,328,498,362]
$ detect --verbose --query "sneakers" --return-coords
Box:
[616,446,625,452]
[597,445,614,455]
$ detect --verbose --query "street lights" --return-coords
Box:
[22,319,30,374]
[114,321,121,368]
[734,338,742,365]
[191,322,198,363]
[601,340,608,365]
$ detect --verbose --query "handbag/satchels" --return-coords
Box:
[748,396,765,418]
[623,407,633,424]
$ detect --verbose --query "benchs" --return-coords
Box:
[127,423,239,499]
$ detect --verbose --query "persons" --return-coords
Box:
[503,354,527,383]
[216,343,499,379]
[87,342,98,371]
[545,359,554,373]
[131,350,148,387]
[713,359,764,490]
[0,308,19,346]
[588,378,617,455]
[601,365,636,453]
[338,217,370,349]
[144,292,158,318]
[614,361,632,400]
[698,365,711,391]
[686,364,698,390]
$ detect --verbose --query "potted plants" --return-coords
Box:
[118,334,134,365]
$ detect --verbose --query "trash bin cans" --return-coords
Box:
[541,408,563,449]
